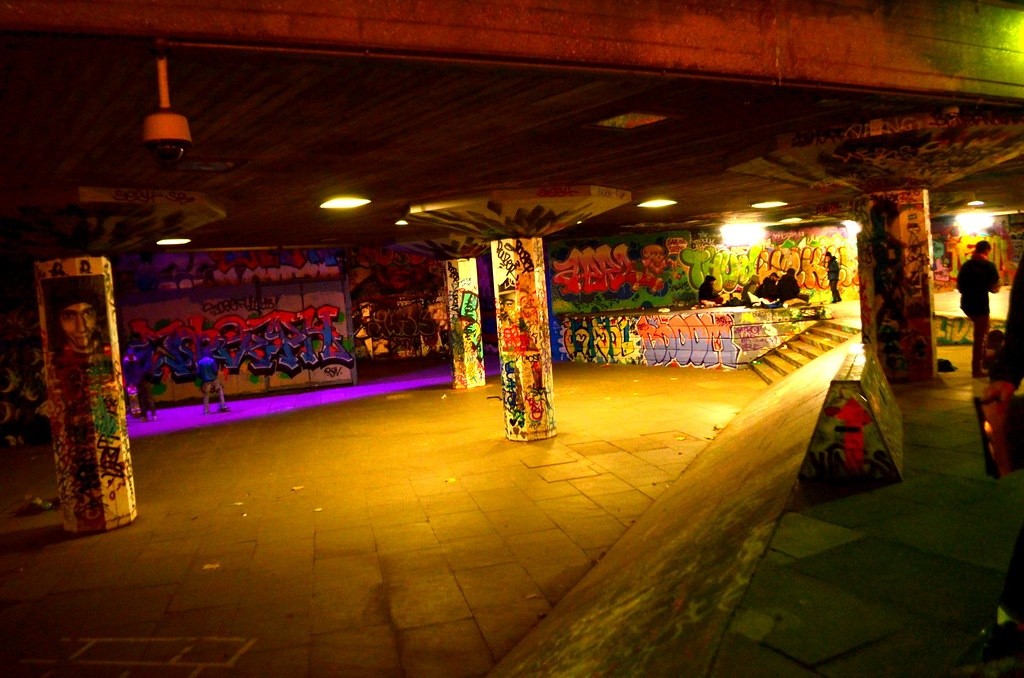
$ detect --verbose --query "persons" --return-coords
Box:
[197,349,229,414]
[741,273,761,301]
[754,272,781,300]
[980,330,1006,373]
[122,347,158,421]
[826,251,842,304]
[698,274,724,304]
[772,268,800,303]
[956,240,1001,378]
[984,248,1024,471]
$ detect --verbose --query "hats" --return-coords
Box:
[825,252,832,256]
[771,271,780,278]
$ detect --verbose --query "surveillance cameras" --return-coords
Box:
[142,113,192,159]
[943,105,959,119]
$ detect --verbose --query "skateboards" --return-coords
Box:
[983,329,1004,375]
[221,404,230,413]
[974,392,1024,480]
[128,385,141,417]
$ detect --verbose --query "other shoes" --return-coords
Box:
[141,417,147,421]
[152,415,158,421]
[970,369,989,378]
[832,298,842,303]
[203,409,210,414]
[220,406,230,412]
